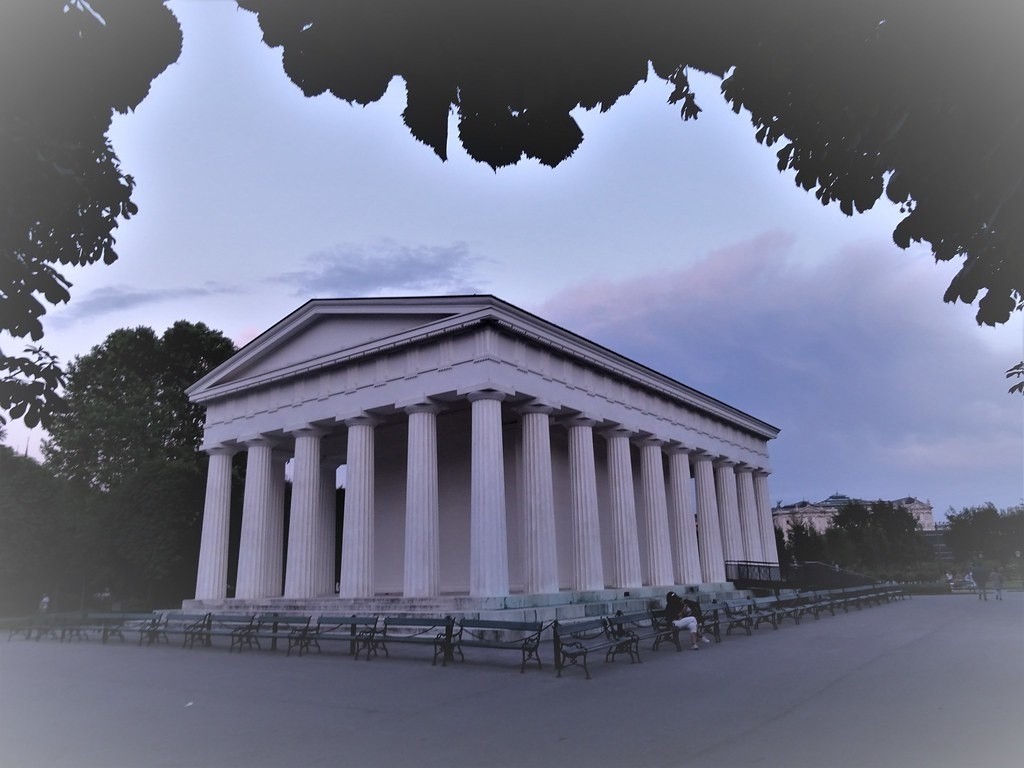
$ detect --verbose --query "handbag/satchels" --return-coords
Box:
[999,575,1004,583]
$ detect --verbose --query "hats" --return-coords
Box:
[667,591,677,600]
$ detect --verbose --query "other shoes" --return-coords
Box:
[691,643,698,649]
[698,636,710,643]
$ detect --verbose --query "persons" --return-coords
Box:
[664,591,710,650]
[972,560,988,601]
[943,568,978,595]
[991,566,1005,600]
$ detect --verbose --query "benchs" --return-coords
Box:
[298,615,378,660]
[190,612,252,651]
[239,614,312,656]
[146,612,206,648]
[552,585,905,679]
[365,616,453,666]
[31,617,72,643]
[105,613,163,646]
[69,612,125,642]
[443,617,542,674]
[7,617,46,640]
